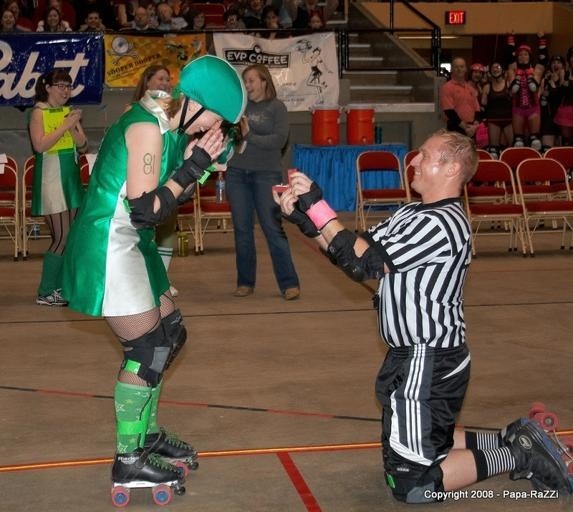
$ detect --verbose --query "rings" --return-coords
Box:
[279,182,283,187]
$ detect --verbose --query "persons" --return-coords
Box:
[119,66,181,300]
[435,27,573,191]
[225,62,302,302]
[25,68,88,306]
[0,1,346,50]
[268,127,573,506]
[53,54,249,489]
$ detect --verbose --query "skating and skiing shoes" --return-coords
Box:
[499,402,573,496]
[111,428,198,507]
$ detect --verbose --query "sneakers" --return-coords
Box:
[234,287,254,296]
[36,288,68,306]
[286,288,300,300]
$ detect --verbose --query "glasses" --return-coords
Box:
[52,84,72,90]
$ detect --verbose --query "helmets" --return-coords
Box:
[178,54,247,125]
[471,63,484,71]
[517,45,531,54]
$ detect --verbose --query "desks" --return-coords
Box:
[292,142,408,211]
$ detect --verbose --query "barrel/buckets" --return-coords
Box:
[346,104,375,145]
[309,105,343,146]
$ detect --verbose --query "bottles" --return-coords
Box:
[215,171,226,203]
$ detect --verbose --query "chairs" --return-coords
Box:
[354,146,573,259]
[0,154,233,262]
